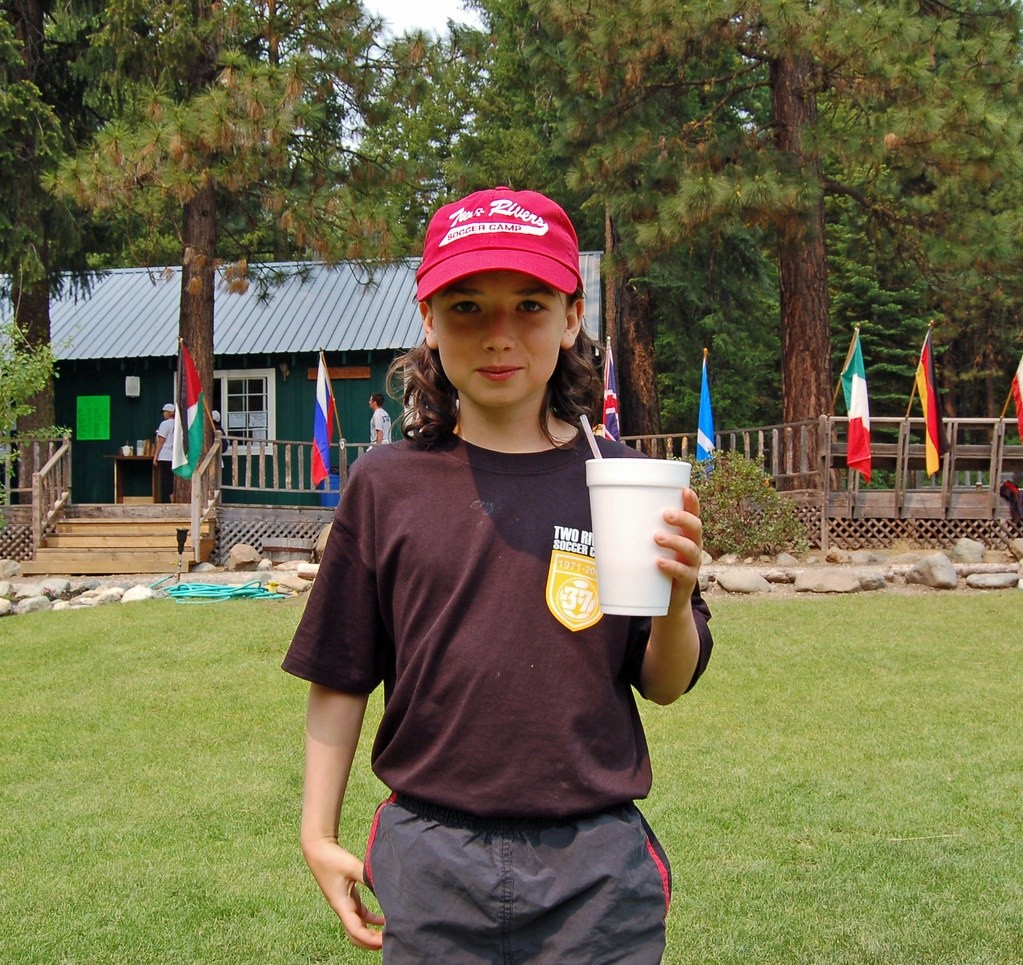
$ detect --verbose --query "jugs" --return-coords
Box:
[121,445,133,457]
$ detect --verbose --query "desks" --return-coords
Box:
[105,455,163,504]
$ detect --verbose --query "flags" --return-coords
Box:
[312,355,334,487]
[697,363,716,480]
[1013,357,1023,442]
[840,334,871,483]
[172,340,205,480]
[603,347,620,442]
[917,332,951,479]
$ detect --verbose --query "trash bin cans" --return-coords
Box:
[318,464,350,507]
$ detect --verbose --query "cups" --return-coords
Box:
[137,440,144,457]
[585,459,691,615]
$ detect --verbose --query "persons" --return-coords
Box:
[369,394,391,448]
[281,186,715,965]
[212,410,228,453]
[154,404,175,503]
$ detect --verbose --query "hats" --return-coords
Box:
[162,403,174,411]
[211,410,220,422]
[415,186,583,302]
